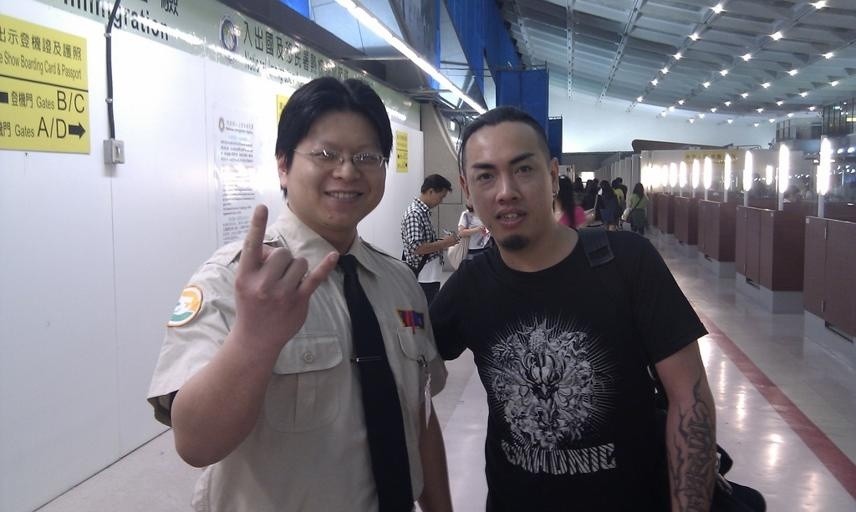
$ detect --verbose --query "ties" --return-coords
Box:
[336,254,413,511]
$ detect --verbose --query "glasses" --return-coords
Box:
[294,149,388,170]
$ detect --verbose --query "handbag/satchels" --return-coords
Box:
[447,236,471,271]
[709,441,766,511]
[583,209,596,223]
[627,215,631,223]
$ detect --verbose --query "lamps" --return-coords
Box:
[335,0,488,115]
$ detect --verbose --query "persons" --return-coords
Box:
[458,173,650,260]
[430,106,716,510]
[402,175,457,306]
[145,77,454,511]
